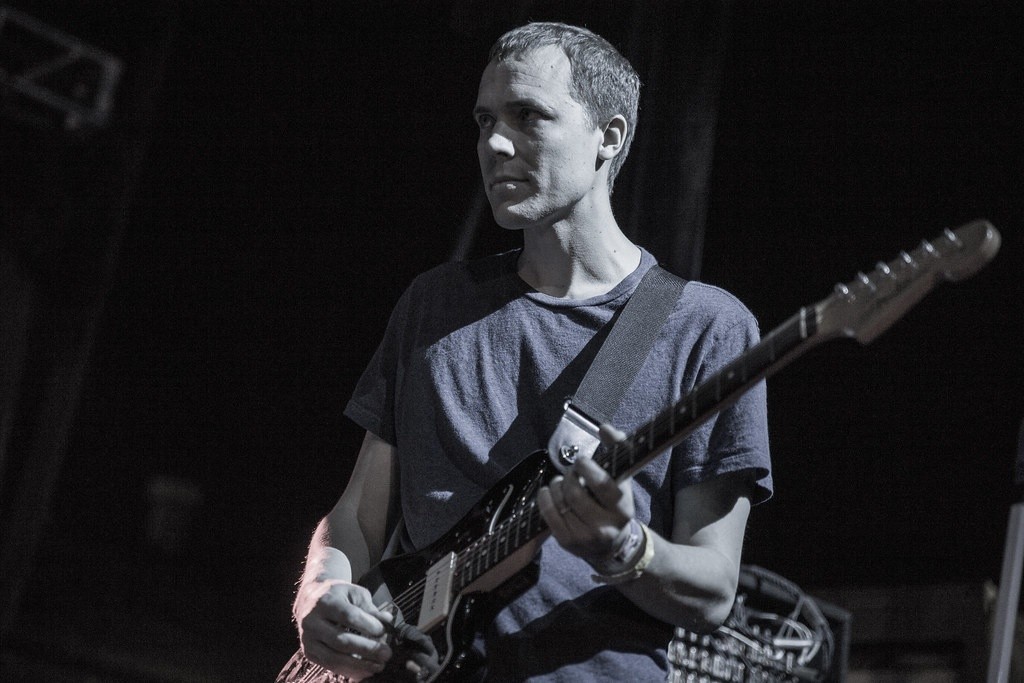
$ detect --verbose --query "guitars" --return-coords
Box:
[272,218,1002,683]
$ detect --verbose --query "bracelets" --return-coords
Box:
[588,518,655,586]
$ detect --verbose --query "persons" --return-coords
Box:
[291,22,775,683]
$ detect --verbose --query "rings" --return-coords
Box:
[560,507,569,516]
